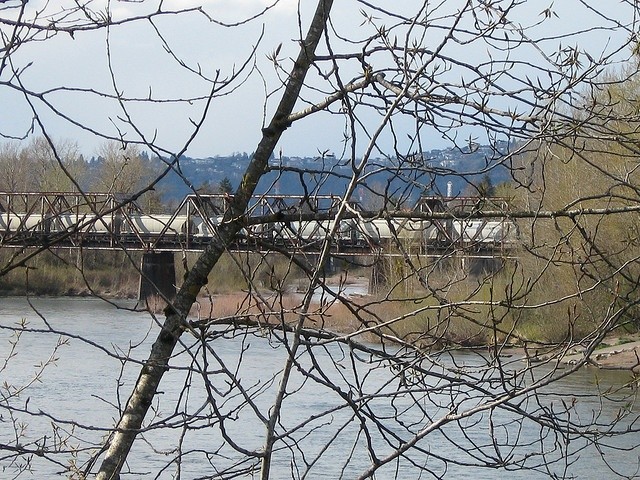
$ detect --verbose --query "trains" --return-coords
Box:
[0,213,516,250]
